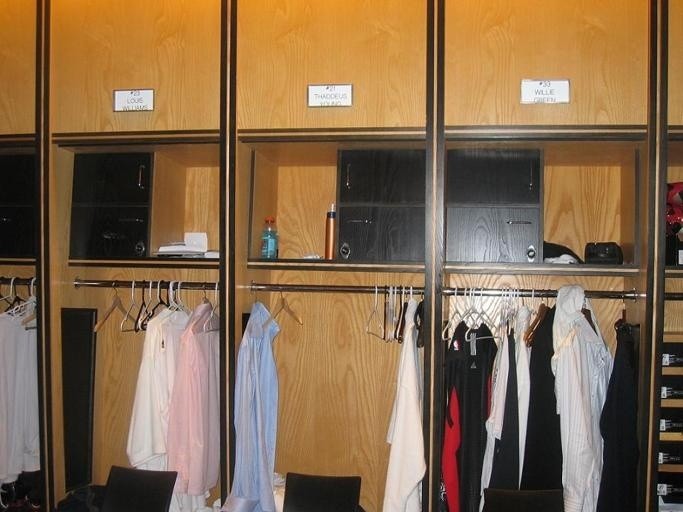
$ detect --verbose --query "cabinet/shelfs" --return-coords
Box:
[0,0,683,512]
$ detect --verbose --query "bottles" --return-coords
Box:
[258,217,276,259]
[324,203,335,260]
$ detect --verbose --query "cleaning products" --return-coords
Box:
[260,215,280,261]
